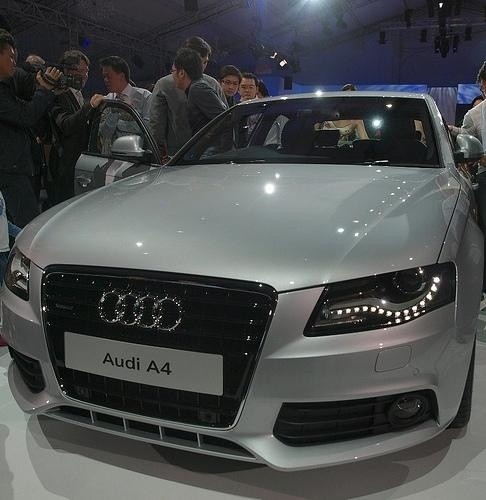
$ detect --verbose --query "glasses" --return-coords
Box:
[220,79,241,87]
[169,69,177,74]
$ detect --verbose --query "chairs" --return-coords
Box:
[276,119,417,163]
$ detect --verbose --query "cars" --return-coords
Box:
[0,90,486,472]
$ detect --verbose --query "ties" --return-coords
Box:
[76,90,85,107]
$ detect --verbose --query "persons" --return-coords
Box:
[314,84,422,148]
[0,27,290,255]
[446,61,486,233]
